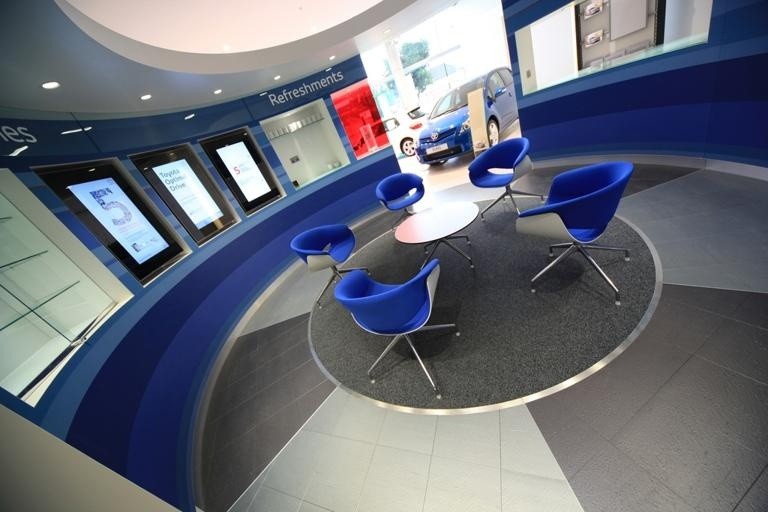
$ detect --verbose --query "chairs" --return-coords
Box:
[468,138,545,222]
[334,258,461,401]
[516,160,636,307]
[290,224,371,309]
[375,172,425,230]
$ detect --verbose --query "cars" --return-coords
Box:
[354,105,430,156]
[413,66,520,165]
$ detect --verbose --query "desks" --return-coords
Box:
[395,200,480,271]
